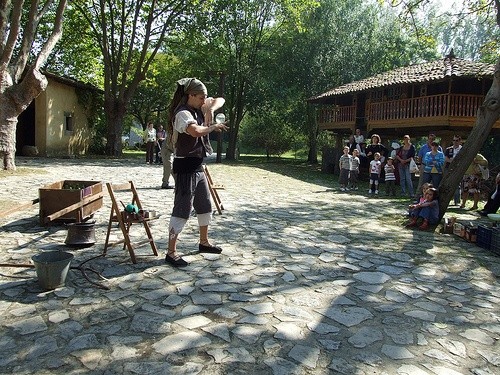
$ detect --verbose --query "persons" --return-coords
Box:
[477,173,500,217]
[396,135,415,197]
[414,134,442,198]
[154,125,166,164]
[384,156,396,197]
[460,149,488,209]
[161,136,174,188]
[144,122,156,165]
[368,152,381,194]
[340,146,352,191]
[407,183,433,218]
[445,135,462,205]
[164,78,229,266]
[350,129,400,181]
[349,149,360,189]
[424,142,445,189]
[406,188,440,230]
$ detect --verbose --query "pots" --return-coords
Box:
[64,220,96,245]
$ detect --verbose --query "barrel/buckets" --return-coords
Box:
[31,249,74,289]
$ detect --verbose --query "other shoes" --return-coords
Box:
[375,190,379,195]
[340,188,345,192]
[345,187,349,191]
[162,184,173,189]
[145,160,164,166]
[368,189,372,194]
[383,191,489,219]
[406,222,416,228]
[165,254,188,267]
[419,224,429,229]
[354,187,358,190]
[350,187,354,190]
[199,243,222,253]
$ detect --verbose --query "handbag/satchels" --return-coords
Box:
[409,157,419,173]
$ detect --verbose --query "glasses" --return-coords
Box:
[452,140,458,142]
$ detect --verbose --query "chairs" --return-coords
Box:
[103,181,158,264]
[201,162,225,215]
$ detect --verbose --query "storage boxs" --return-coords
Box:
[452,220,500,256]
